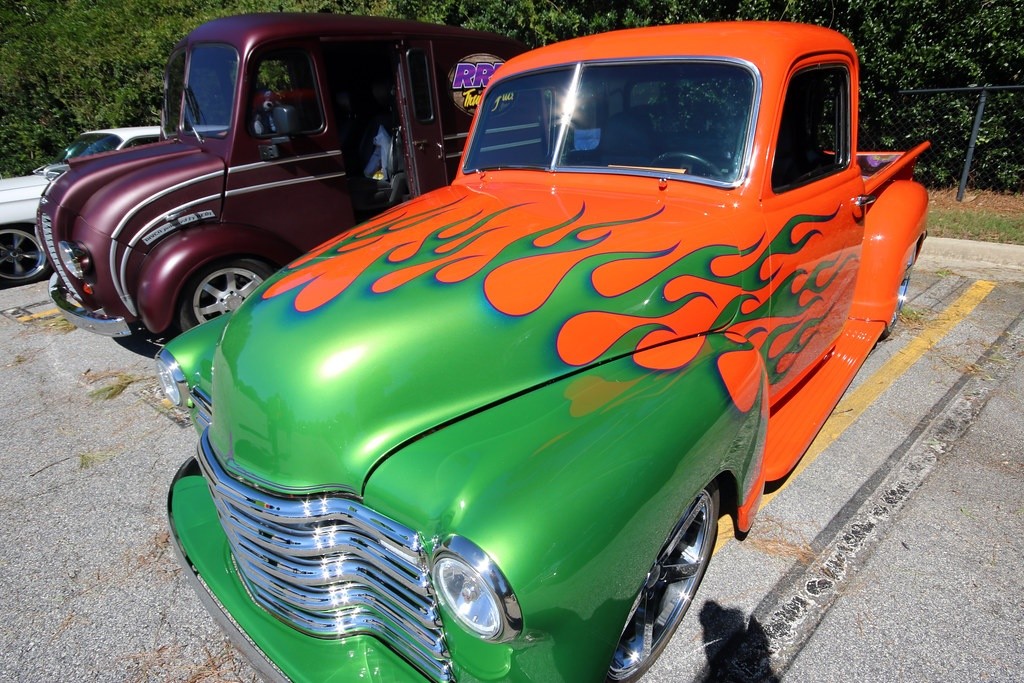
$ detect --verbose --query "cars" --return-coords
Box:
[0,125,228,289]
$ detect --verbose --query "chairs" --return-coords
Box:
[344,120,406,213]
[600,109,656,166]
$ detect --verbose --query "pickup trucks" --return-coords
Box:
[153,18,931,682]
[41,12,732,346]
